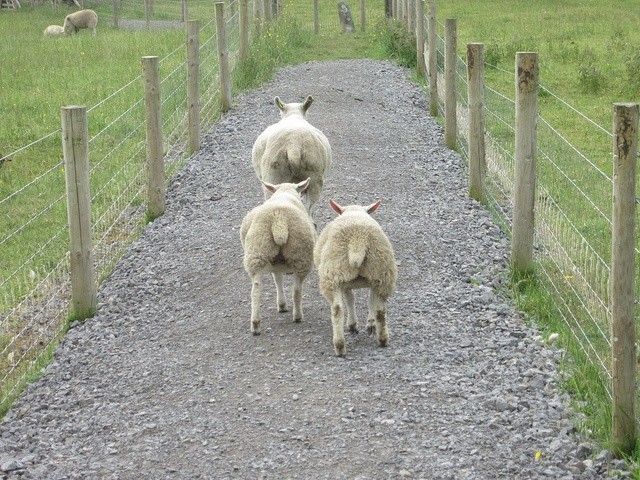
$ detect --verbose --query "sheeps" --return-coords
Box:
[313,197,398,359]
[42,25,64,38]
[62,8,99,35]
[251,94,332,237]
[238,179,318,337]
